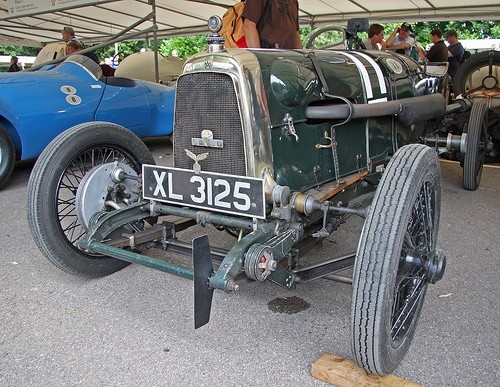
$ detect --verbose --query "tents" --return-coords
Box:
[426,38,500,54]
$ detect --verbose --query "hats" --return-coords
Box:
[444,30,457,38]
[401,23,411,31]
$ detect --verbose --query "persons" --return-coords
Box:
[8,56,20,73]
[31,41,81,68]
[100,55,119,68]
[62,26,84,50]
[443,30,465,78]
[386,22,414,55]
[407,34,418,62]
[240,0,302,49]
[416,28,448,62]
[359,23,387,50]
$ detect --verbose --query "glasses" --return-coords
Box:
[64,28,68,34]
[400,29,409,33]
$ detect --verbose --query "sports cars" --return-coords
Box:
[0,55,177,190]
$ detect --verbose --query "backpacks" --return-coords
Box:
[455,43,472,66]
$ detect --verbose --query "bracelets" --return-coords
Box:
[392,32,396,35]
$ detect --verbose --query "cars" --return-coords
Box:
[24,15,496,376]
